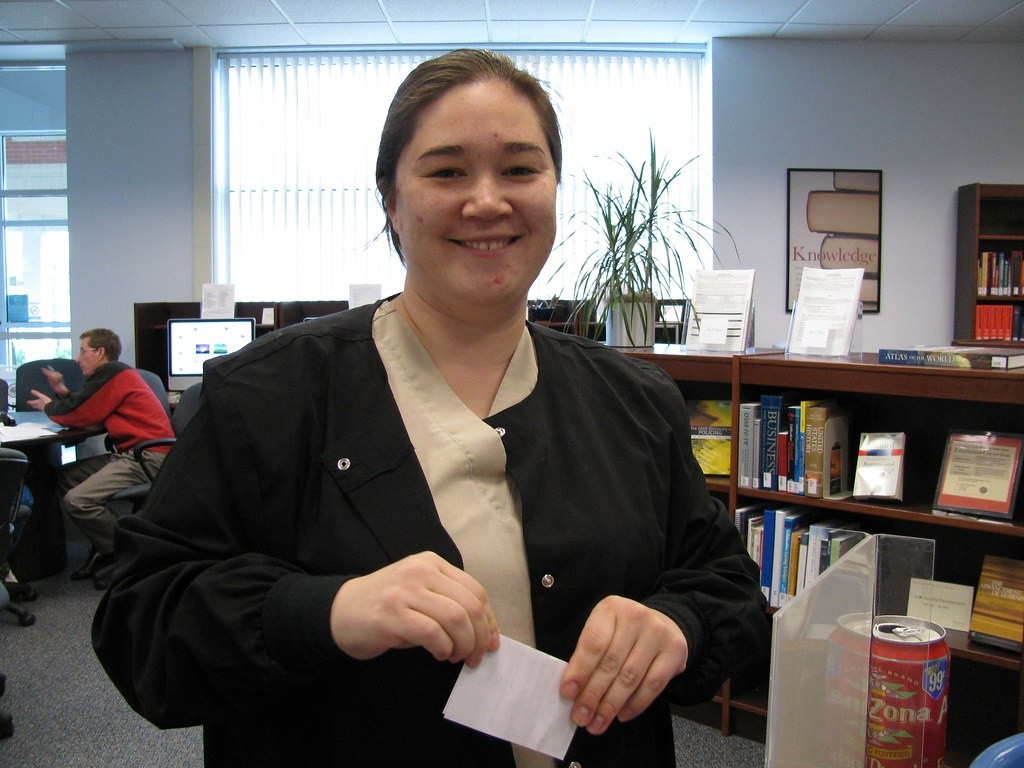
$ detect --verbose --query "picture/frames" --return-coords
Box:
[785,168,883,312]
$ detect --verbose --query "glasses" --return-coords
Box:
[80,346,97,356]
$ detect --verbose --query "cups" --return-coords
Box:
[969,732,1024,768]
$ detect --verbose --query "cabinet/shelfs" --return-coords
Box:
[602,342,1024,768]
[130,300,349,393]
[952,183,1024,347]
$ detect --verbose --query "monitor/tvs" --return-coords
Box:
[166,318,256,391]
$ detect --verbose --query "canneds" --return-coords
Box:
[825,612,950,768]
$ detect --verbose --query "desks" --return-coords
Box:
[0,411,107,583]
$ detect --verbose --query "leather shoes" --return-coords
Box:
[71,549,104,581]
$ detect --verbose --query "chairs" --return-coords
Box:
[137,368,172,415]
[0,447,36,627]
[109,381,204,514]
[15,359,86,412]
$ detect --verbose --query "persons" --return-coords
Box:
[92,48,774,768]
[13,328,177,580]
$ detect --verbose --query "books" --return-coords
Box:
[878,345,1024,371]
[976,250,1024,344]
[679,394,1024,656]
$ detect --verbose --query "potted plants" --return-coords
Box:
[528,293,568,321]
[540,125,742,351]
